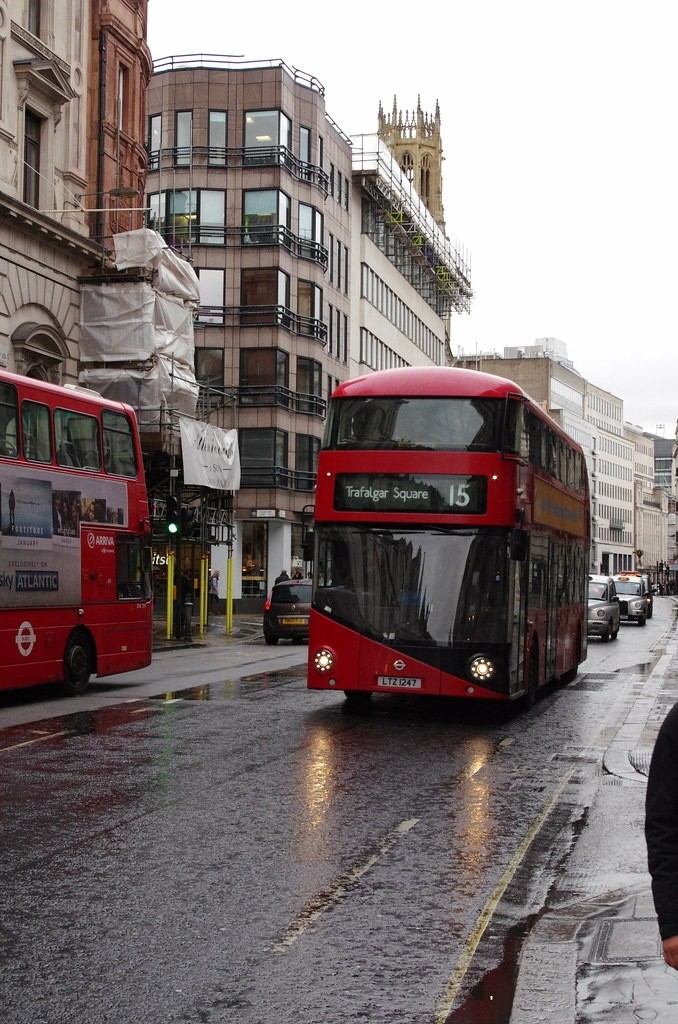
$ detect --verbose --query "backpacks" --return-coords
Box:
[208,576,219,591]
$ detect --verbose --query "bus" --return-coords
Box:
[0,368,153,698]
[299,364,593,712]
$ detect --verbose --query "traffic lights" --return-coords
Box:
[165,496,180,536]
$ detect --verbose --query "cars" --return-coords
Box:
[262,578,331,647]
[588,574,621,643]
[611,576,649,627]
[620,571,657,620]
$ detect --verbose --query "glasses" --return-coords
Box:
[63,506,67,509]
[89,509,95,515]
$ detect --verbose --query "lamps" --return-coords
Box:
[73,186,141,207]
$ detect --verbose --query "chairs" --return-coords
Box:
[0,429,119,475]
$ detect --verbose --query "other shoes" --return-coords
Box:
[214,610,222,615]
[209,612,213,615]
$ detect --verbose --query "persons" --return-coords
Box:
[274,570,291,586]
[292,566,304,580]
[178,575,195,643]
[208,571,222,615]
[54,497,79,538]
[644,703,678,971]
[83,500,123,524]
